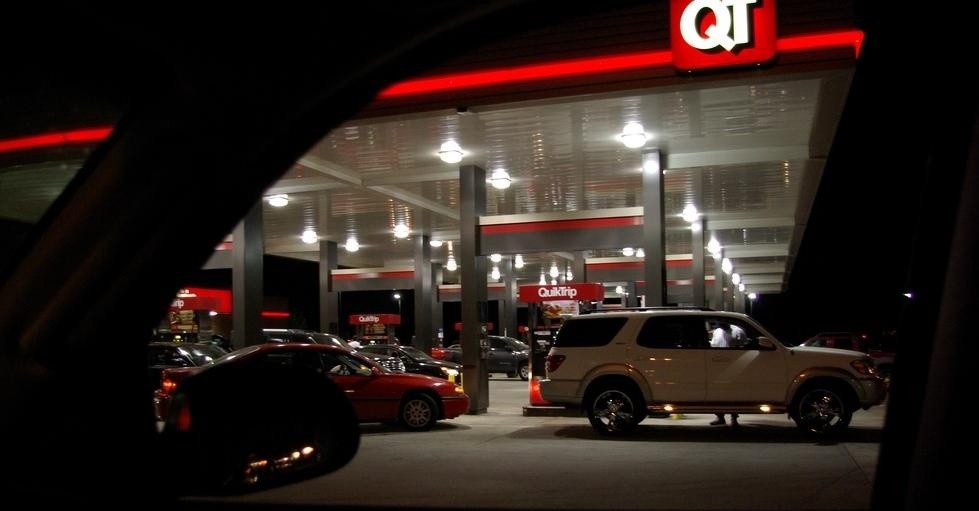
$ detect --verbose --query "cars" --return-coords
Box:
[157,332,232,353]
[359,344,464,384]
[146,340,227,377]
[152,343,472,434]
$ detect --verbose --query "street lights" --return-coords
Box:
[394,293,402,314]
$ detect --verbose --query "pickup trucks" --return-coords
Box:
[798,331,895,366]
[431,335,529,383]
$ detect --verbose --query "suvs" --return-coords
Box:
[538,308,887,439]
[251,329,381,374]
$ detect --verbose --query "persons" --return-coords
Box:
[710,321,747,425]
[188,323,201,343]
